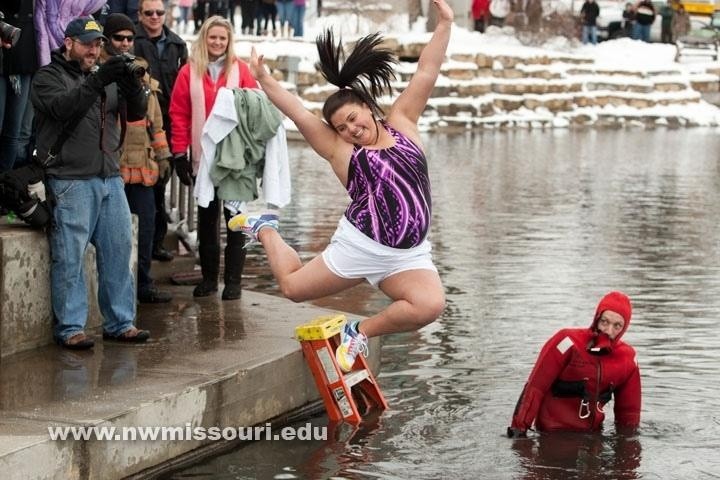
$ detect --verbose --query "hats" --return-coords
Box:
[65,17,110,44]
[103,13,136,39]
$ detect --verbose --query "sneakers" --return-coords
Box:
[335,319,370,373]
[61,334,95,350]
[138,283,173,303]
[227,209,280,249]
[102,328,150,343]
[153,247,173,261]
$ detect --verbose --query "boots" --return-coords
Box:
[193,243,219,297]
[221,247,247,300]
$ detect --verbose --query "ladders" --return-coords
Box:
[294,314,388,423]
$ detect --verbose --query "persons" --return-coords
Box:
[168,16,264,302]
[227,0,456,374]
[30,16,151,351]
[469,0,692,46]
[0,1,110,230]
[134,1,189,260]
[172,1,307,41]
[506,292,643,442]
[101,12,175,304]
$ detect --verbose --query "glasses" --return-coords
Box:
[112,34,133,42]
[144,9,165,16]
[73,39,104,49]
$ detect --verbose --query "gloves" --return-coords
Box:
[174,154,195,185]
[96,57,126,86]
[117,77,143,98]
[158,159,171,184]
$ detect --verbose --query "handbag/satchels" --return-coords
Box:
[1,155,55,230]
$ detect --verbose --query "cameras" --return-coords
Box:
[113,52,145,81]
[1,13,22,45]
